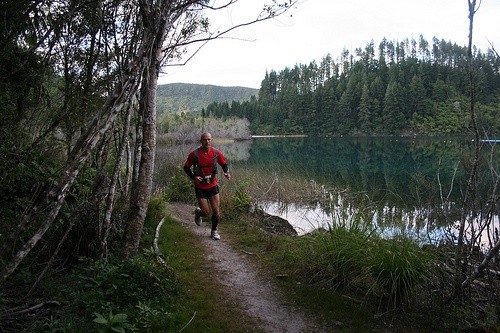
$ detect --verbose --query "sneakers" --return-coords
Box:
[211,230,220,240]
[195,207,203,226]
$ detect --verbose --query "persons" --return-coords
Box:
[183,132,232,240]
